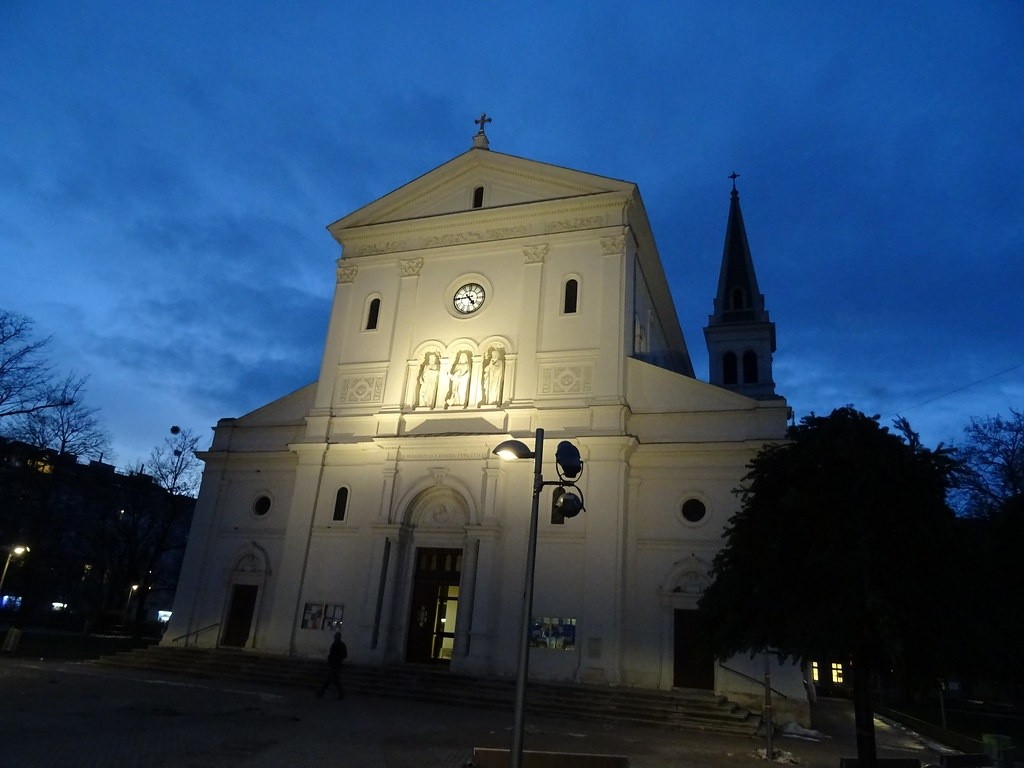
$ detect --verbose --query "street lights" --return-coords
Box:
[492,428,587,768]
[122,584,141,625]
[0,544,26,590]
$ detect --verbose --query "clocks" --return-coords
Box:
[453,283,485,314]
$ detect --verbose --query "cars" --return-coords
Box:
[83,610,143,642]
[940,698,1024,733]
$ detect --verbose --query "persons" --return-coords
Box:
[315,631,347,701]
